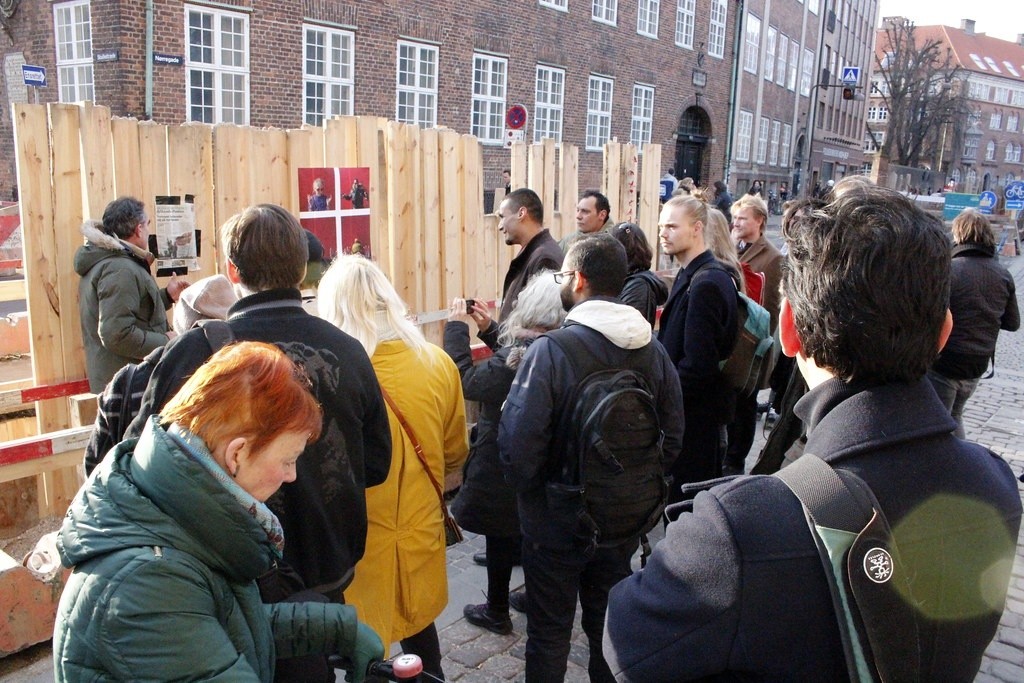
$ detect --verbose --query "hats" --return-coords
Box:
[173,274,237,336]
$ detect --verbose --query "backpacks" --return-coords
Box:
[740,262,766,307]
[536,329,674,562]
[718,291,775,401]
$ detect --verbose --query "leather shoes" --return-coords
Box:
[473,552,487,565]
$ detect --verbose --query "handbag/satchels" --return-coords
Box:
[442,517,463,546]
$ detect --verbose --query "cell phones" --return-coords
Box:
[466,299,475,314]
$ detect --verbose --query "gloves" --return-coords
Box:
[327,619,385,683]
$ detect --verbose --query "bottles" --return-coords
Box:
[392,653,425,683]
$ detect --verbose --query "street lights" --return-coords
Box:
[938,83,952,172]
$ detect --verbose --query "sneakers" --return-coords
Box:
[464,600,513,635]
[509,591,527,613]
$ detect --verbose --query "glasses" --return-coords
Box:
[553,270,590,284]
[314,188,324,191]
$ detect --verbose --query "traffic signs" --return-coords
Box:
[21,64,47,87]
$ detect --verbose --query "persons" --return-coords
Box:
[72,197,191,393]
[654,175,874,533]
[598,173,1023,683]
[82,277,240,476]
[124,204,391,612]
[659,167,678,205]
[49,341,384,683]
[749,180,762,197]
[306,178,334,212]
[503,169,511,196]
[316,256,470,683]
[297,227,331,319]
[926,204,1019,439]
[712,180,733,225]
[341,178,368,208]
[493,229,686,682]
[442,189,670,632]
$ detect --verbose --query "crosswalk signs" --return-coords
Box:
[841,66,860,83]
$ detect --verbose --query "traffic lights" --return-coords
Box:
[842,84,856,101]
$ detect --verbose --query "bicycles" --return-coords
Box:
[767,189,795,216]
